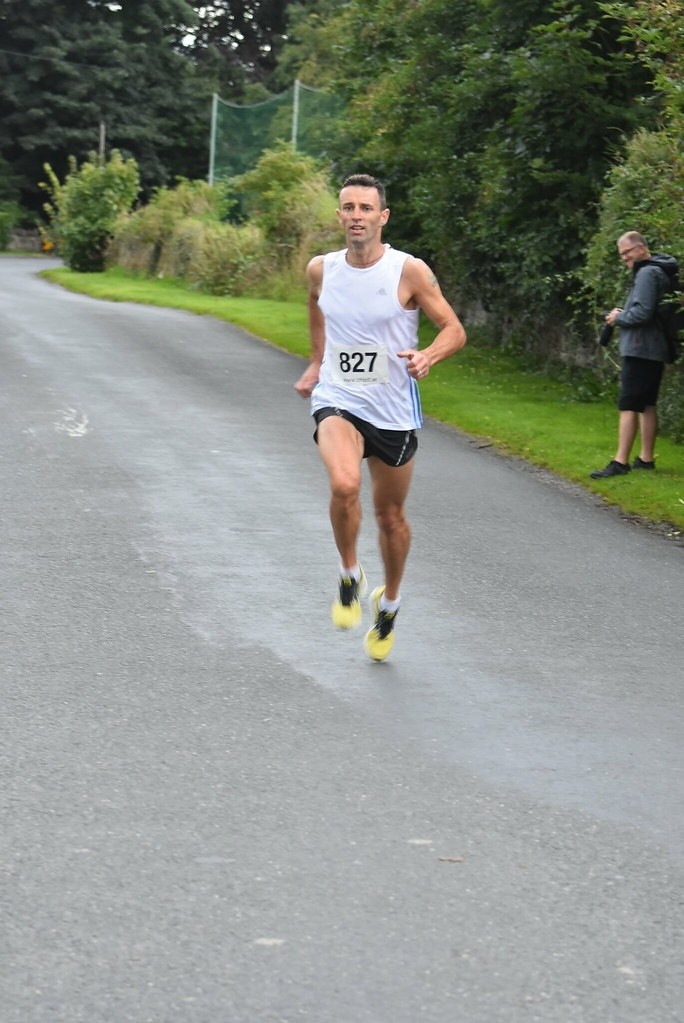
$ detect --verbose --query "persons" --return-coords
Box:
[590,231,678,479]
[293,175,466,663]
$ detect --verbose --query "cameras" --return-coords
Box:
[600,310,621,345]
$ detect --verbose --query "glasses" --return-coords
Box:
[620,246,639,255]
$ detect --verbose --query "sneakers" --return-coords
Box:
[630,456,656,469]
[365,586,401,663]
[332,561,368,630]
[590,460,631,478]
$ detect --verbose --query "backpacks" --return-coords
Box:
[658,266,680,363]
